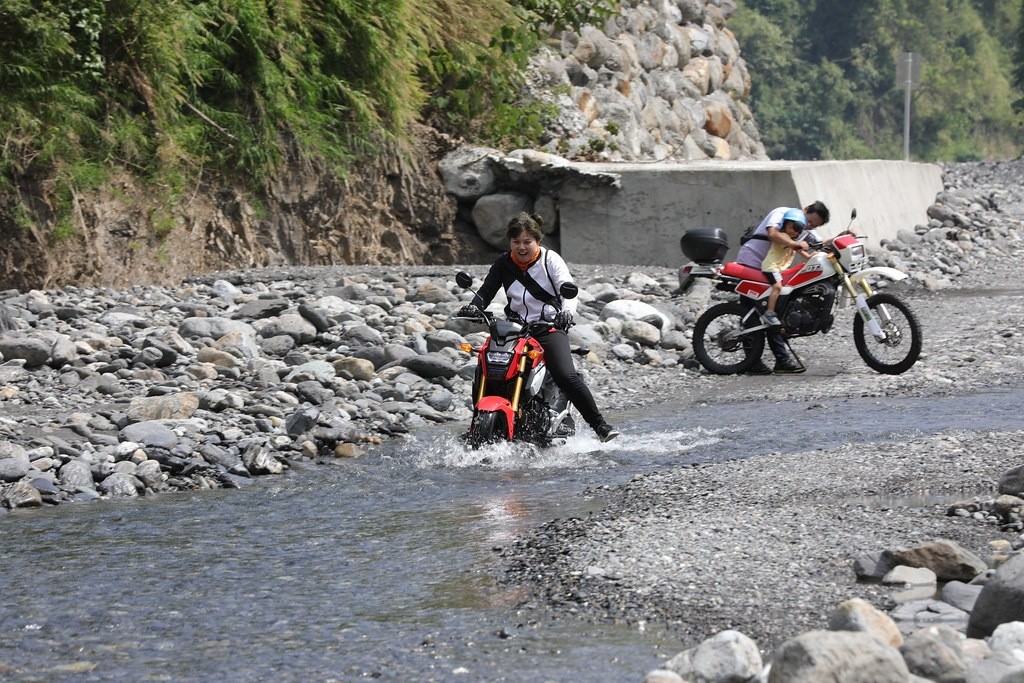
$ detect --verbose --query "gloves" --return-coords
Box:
[554,311,572,329]
[457,305,478,323]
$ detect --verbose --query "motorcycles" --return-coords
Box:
[670,207,924,376]
[449,271,591,449]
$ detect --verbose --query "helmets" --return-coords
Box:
[783,209,805,225]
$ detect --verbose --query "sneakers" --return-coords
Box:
[748,360,772,374]
[774,360,805,373]
[596,424,619,443]
[458,431,469,443]
[761,311,781,325]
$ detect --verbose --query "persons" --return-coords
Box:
[762,209,818,326]
[736,200,858,376]
[457,212,620,443]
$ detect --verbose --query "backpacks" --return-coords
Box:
[741,222,771,245]
